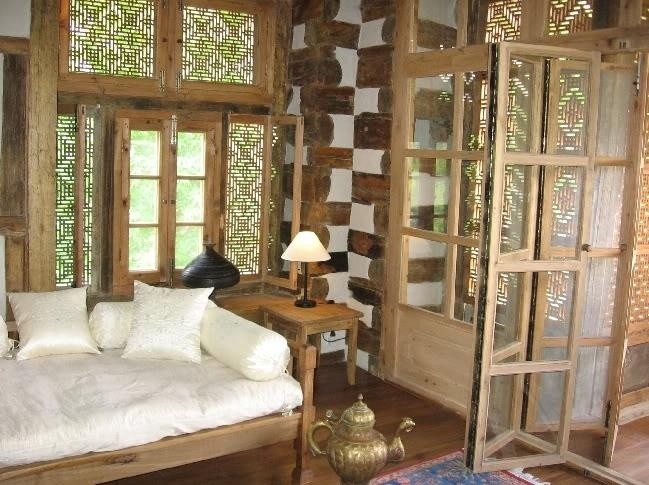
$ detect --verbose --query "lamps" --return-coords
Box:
[281,228,331,308]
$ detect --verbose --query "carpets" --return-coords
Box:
[367,448,552,485]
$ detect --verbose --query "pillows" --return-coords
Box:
[0,280,291,383]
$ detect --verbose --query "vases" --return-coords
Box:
[181,242,241,289]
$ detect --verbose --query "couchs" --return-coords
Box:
[0,319,317,484]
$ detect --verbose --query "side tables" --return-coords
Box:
[260,302,364,385]
